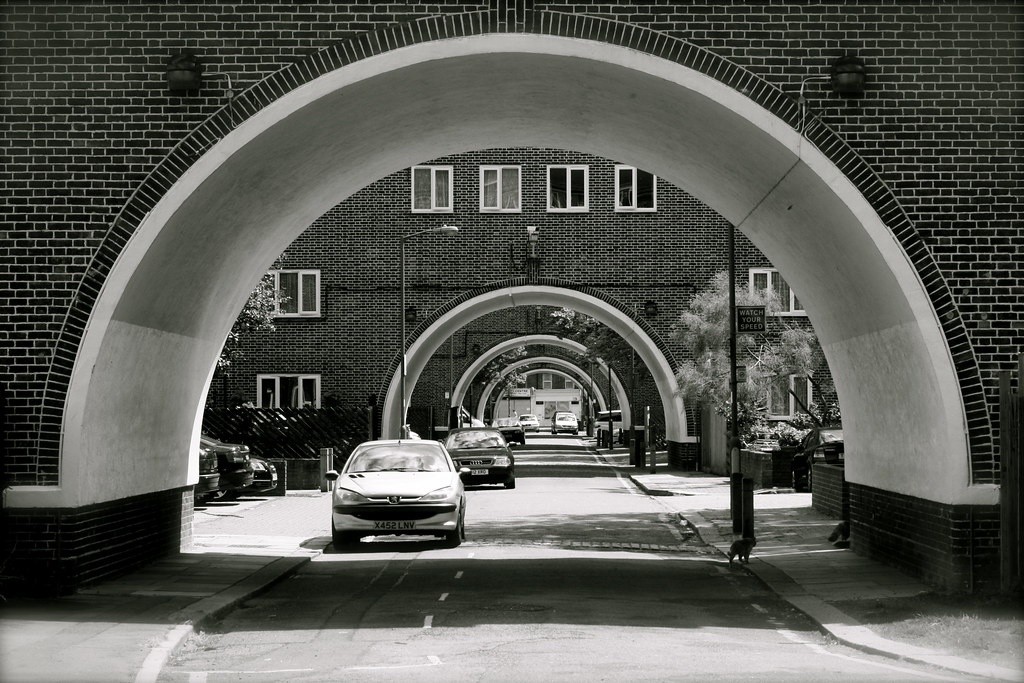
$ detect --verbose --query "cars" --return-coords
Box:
[492,418,525,444]
[519,414,539,432]
[439,427,516,489]
[790,426,843,492]
[550,411,579,435]
[195,434,278,508]
[323,438,465,547]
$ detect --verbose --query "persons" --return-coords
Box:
[513,410,518,417]
[402,424,421,440]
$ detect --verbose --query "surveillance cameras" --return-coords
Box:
[526,225,540,232]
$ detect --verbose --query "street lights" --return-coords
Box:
[399,225,459,438]
